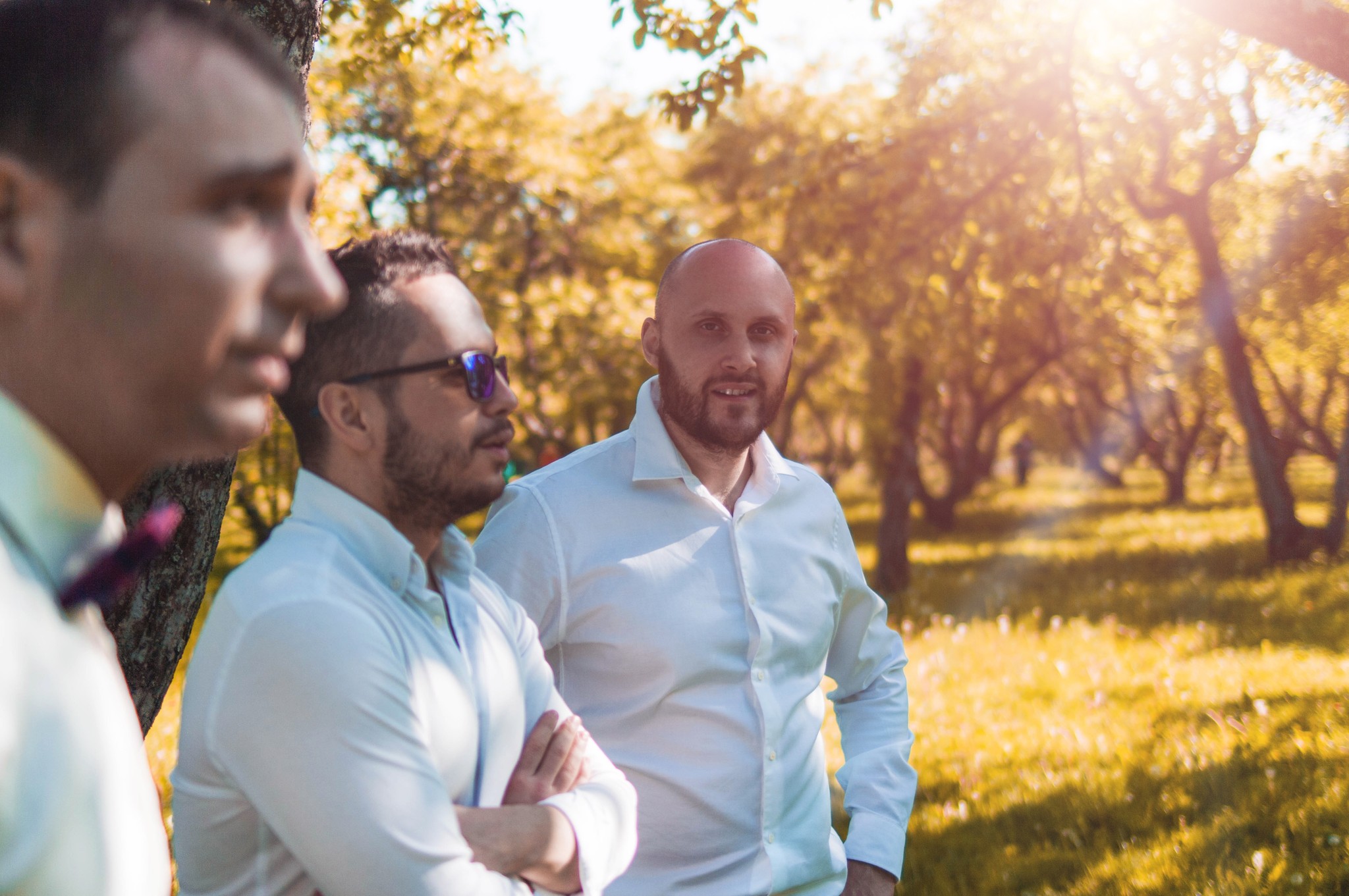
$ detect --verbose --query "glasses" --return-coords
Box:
[338,351,512,402]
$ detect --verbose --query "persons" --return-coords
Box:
[471,235,916,896]
[0,0,348,896]
[167,222,639,896]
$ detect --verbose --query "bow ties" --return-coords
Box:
[56,500,184,615]
